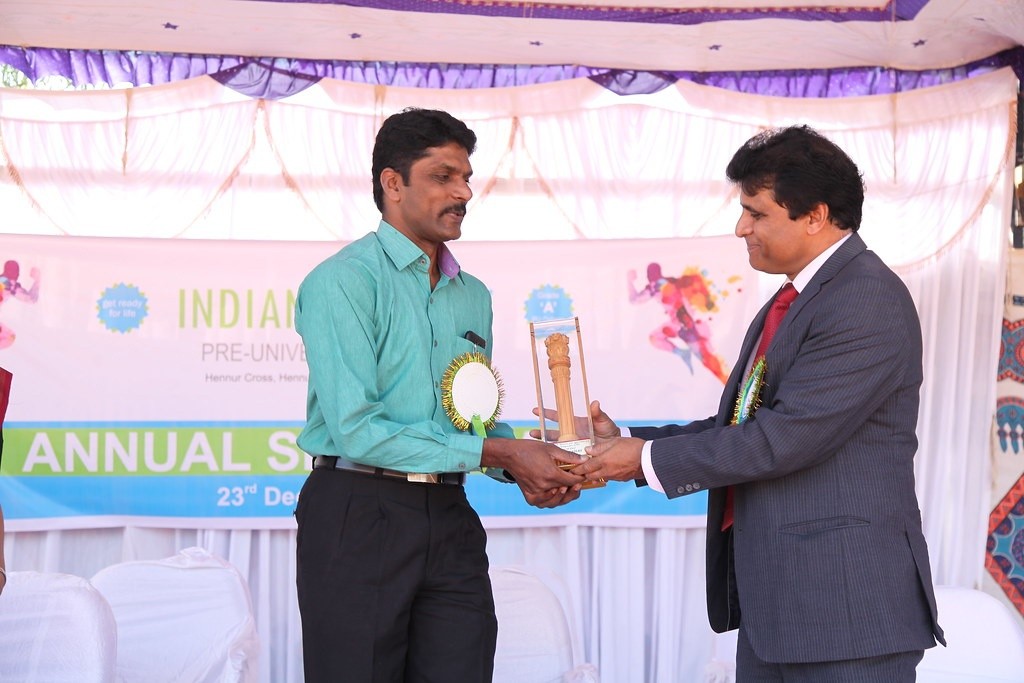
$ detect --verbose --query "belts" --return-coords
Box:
[315,455,467,485]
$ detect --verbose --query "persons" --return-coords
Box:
[529,124,947,683]
[295,108,589,683]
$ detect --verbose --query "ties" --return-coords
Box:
[721,283,799,531]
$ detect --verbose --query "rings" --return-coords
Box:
[599,478,604,483]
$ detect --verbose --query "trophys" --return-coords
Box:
[529,317,606,490]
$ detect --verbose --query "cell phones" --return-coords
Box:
[465,331,486,350]
